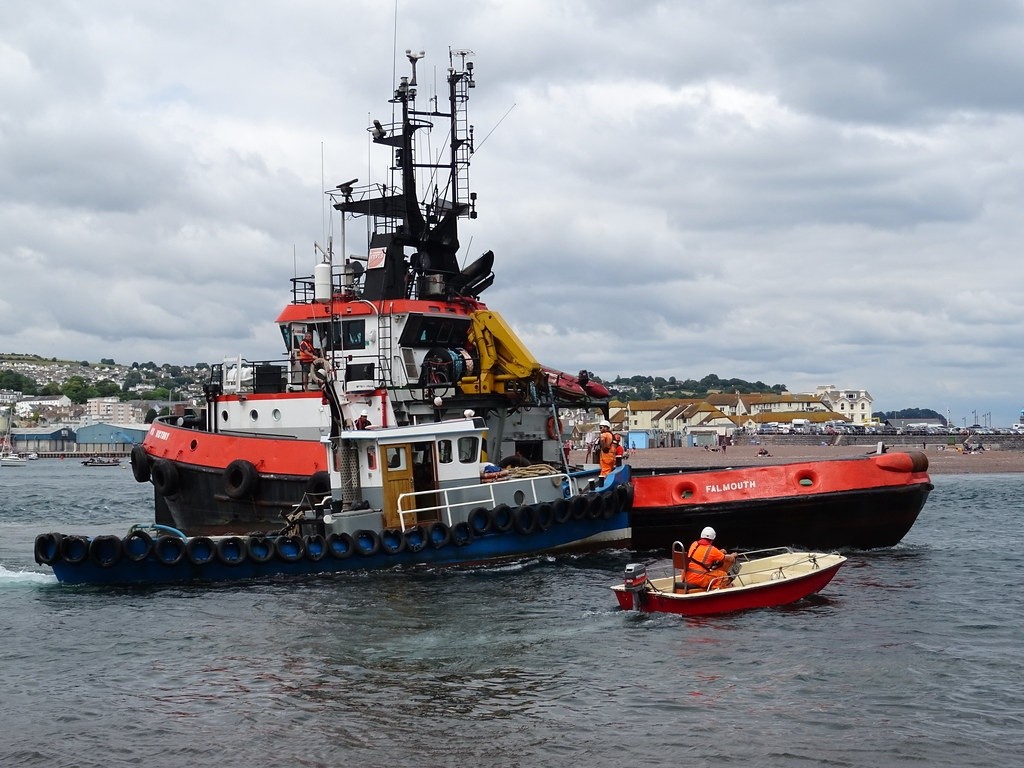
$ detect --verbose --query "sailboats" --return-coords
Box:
[1,407,19,460]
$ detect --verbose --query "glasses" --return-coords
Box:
[599,427,602,429]
[362,416,367,417]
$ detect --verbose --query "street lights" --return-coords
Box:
[962,411,992,427]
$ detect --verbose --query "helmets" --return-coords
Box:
[700,526,716,540]
[599,420,611,428]
[361,409,368,416]
[614,433,621,440]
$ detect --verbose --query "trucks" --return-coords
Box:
[792,418,810,435]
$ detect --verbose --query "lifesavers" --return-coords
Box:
[309,357,332,384]
[150,460,179,497]
[130,444,151,483]
[546,415,564,441]
[32,478,636,571]
[221,458,259,502]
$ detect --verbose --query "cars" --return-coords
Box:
[759,421,792,434]
[810,420,1024,434]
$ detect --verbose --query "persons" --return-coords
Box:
[623,445,637,457]
[90,458,115,463]
[614,434,624,466]
[759,448,768,454]
[597,420,617,480]
[299,331,318,392]
[935,444,944,451]
[962,443,985,452]
[703,444,727,454]
[572,444,573,450]
[564,438,570,464]
[922,441,926,449]
[574,445,577,451]
[354,409,371,430]
[681,525,738,588]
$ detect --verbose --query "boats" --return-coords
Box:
[28,454,38,459]
[601,549,851,619]
[536,365,612,400]
[0,454,27,465]
[33,40,934,585]
[83,457,121,467]
